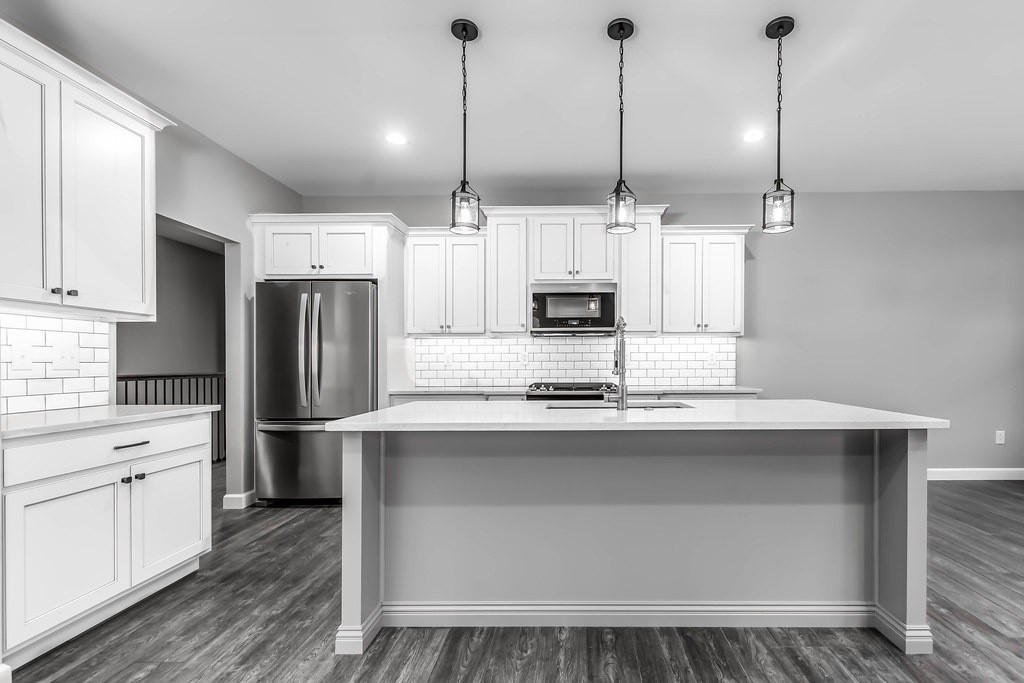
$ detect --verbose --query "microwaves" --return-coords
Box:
[530,284,616,337]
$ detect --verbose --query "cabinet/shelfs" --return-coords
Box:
[403,227,486,336]
[0,405,212,672]
[661,224,756,338]
[248,213,408,279]
[0,18,178,324]
[478,205,671,336]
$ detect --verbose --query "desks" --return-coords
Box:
[326,400,950,654]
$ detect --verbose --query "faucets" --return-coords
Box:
[611,315,627,392]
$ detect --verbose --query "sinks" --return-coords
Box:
[545,400,693,410]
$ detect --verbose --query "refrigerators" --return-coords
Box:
[253,280,378,499]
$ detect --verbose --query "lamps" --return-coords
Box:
[449,19,480,236]
[607,18,637,235]
[762,16,795,234]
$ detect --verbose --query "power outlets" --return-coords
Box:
[996,431,1005,445]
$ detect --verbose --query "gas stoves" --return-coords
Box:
[525,381,620,401]
